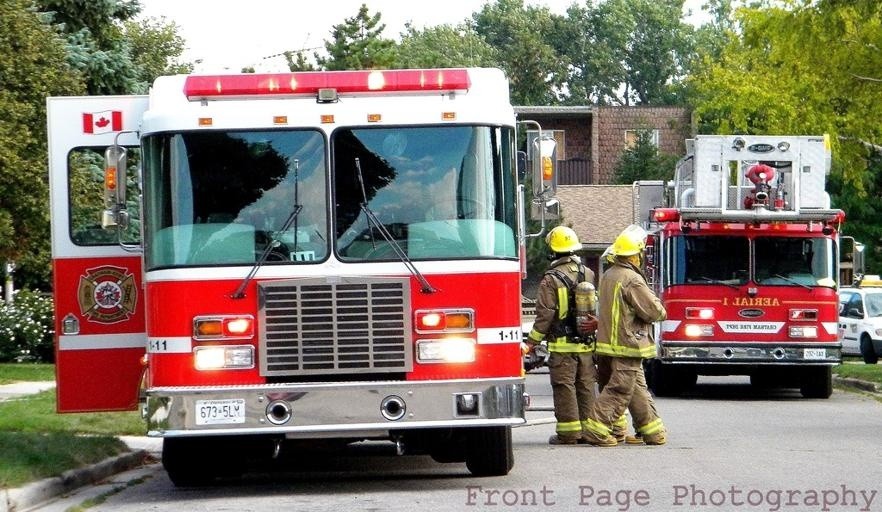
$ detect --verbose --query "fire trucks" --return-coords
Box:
[638,133,860,398]
[43,73,560,487]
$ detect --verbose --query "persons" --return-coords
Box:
[524,223,668,446]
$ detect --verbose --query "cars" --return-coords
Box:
[837,274,881,366]
[521,312,550,373]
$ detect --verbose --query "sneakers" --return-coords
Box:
[550,429,666,446]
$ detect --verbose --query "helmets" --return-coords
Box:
[614,225,647,257]
[546,226,582,253]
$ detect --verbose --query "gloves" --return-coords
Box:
[530,344,548,369]
